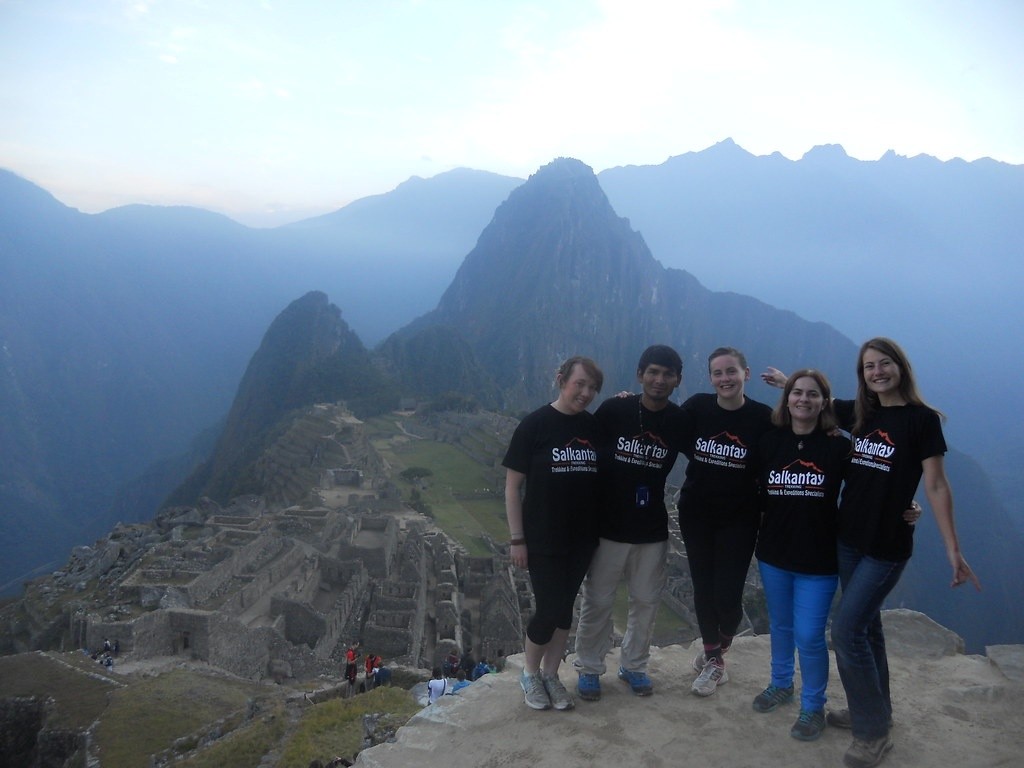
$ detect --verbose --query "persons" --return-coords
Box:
[501,357,606,712]
[573,344,690,701]
[759,336,980,768]
[754,368,923,742]
[345,640,392,696]
[615,345,843,696]
[92,638,120,671]
[428,646,506,707]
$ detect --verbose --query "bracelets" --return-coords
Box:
[510,538,527,544]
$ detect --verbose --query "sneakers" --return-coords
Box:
[826,707,896,730]
[790,708,830,741]
[753,684,796,712]
[616,665,655,696]
[541,674,575,710]
[690,655,731,697]
[842,732,895,768]
[584,671,603,702]
[692,643,732,673]
[518,666,551,710]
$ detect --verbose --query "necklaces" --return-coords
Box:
[639,394,669,467]
[797,440,804,450]
